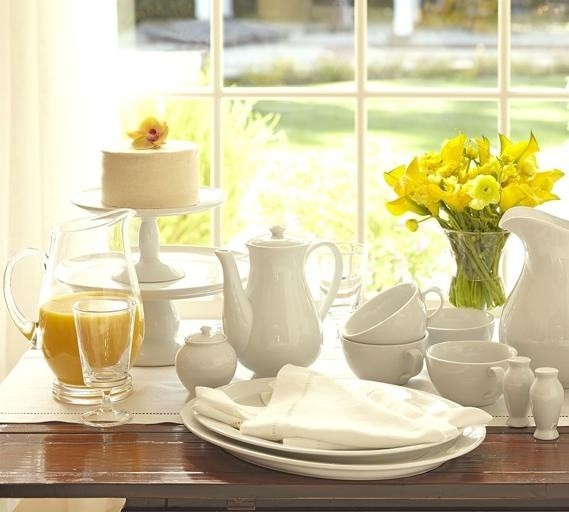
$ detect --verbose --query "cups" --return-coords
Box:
[429,307,495,341]
[343,283,444,385]
[426,340,518,406]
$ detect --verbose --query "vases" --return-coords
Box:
[440,230,511,308]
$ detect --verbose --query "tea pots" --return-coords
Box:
[498,205,568,387]
[3,209,143,406]
[215,225,343,375]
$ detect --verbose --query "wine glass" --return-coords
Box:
[75,296,136,428]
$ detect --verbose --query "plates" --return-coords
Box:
[180,375,488,481]
[78,185,222,282]
[60,248,248,368]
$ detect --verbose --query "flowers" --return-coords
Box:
[383,131,563,312]
